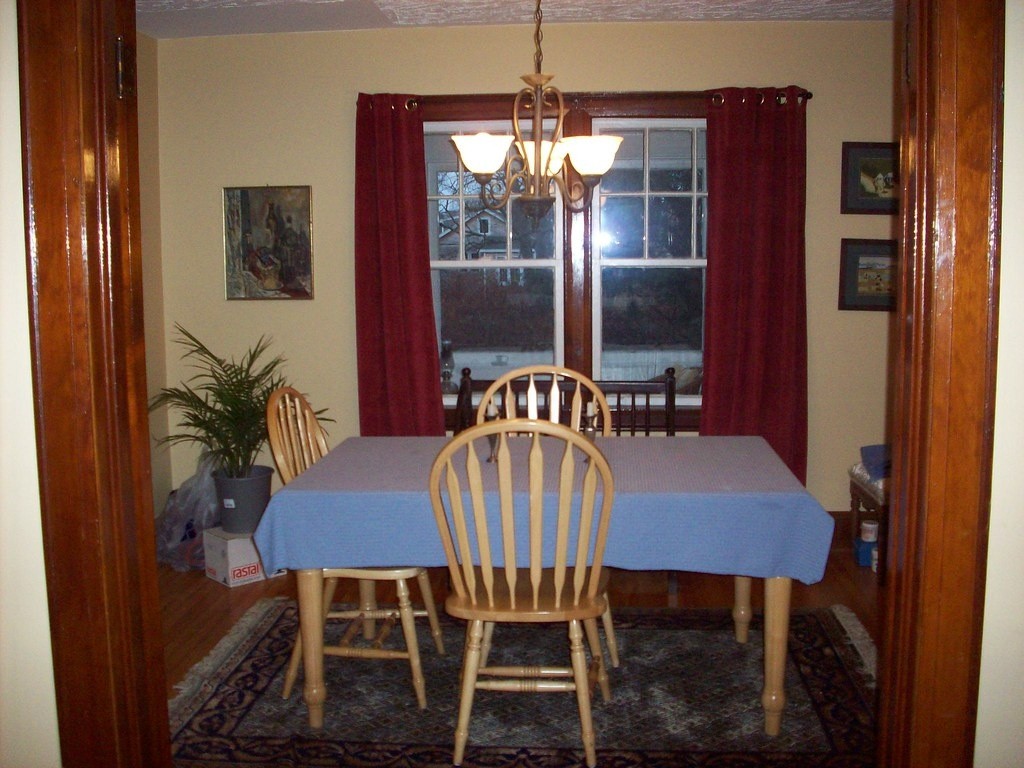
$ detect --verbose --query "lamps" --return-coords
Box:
[452,0,623,230]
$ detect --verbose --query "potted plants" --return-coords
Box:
[145,321,337,534]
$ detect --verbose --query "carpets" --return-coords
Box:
[165,596,879,768]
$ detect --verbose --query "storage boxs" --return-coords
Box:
[203,526,288,589]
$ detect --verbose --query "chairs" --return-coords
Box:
[475,365,619,669]
[267,387,444,710]
[429,419,612,768]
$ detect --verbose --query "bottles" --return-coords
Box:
[861,520,878,542]
[872,548,878,573]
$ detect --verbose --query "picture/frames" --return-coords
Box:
[222,185,314,301]
[841,140,902,215]
[838,237,898,311]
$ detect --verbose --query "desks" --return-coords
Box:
[252,437,835,737]
